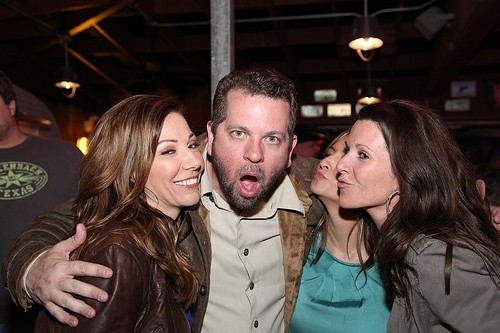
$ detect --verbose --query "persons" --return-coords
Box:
[0,70,85,333]
[1,65,500,333]
[337,99,500,333]
[289,129,392,333]
[34,95,206,333]
